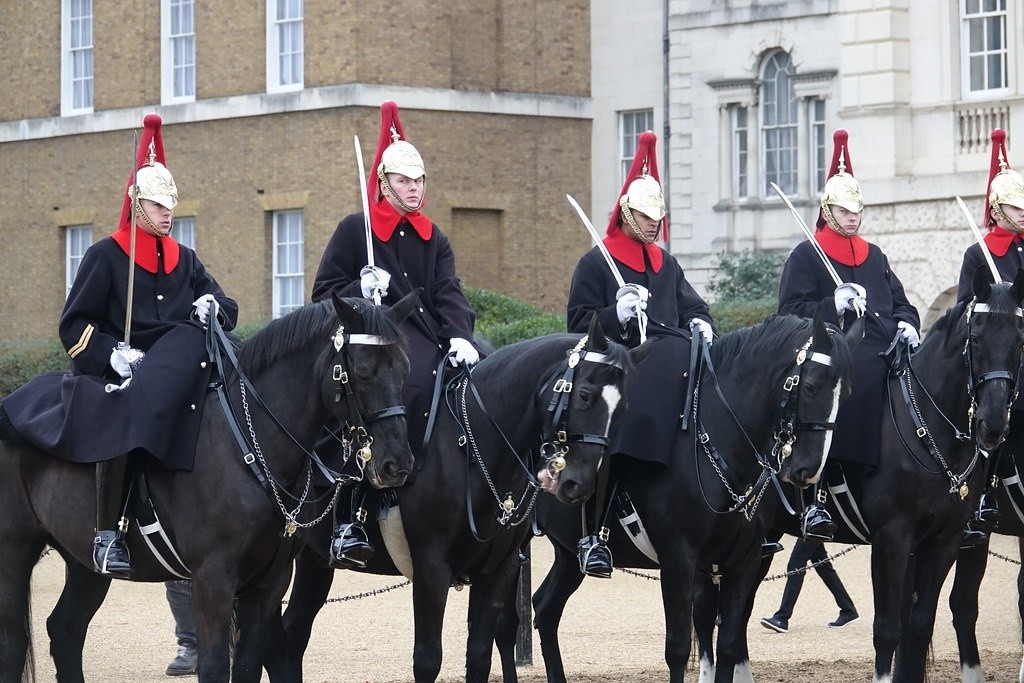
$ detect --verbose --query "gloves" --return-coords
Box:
[898,321,919,348]
[194,294,221,324]
[834,288,859,317]
[689,317,714,346]
[109,351,132,377]
[361,273,387,301]
[447,337,479,366]
[616,293,647,325]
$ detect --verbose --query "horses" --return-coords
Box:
[694,261,1023,683]
[0,285,424,683]
[531,300,867,683]
[266,309,658,683]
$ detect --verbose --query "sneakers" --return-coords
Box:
[829,611,859,629]
[166,640,199,675]
[760,617,789,634]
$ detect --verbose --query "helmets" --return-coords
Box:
[366,100,427,210]
[820,128,864,236]
[606,130,668,244]
[119,113,178,237]
[983,128,1024,234]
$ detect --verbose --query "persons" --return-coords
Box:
[957,171,1024,549]
[166,581,239,676]
[761,537,861,633]
[778,171,921,537]
[0,160,238,578]
[565,174,719,577]
[311,139,482,565]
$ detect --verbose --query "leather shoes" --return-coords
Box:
[960,529,988,547]
[968,494,1001,520]
[517,548,530,566]
[578,535,614,575]
[799,506,837,545]
[94,530,134,574]
[329,527,372,570]
